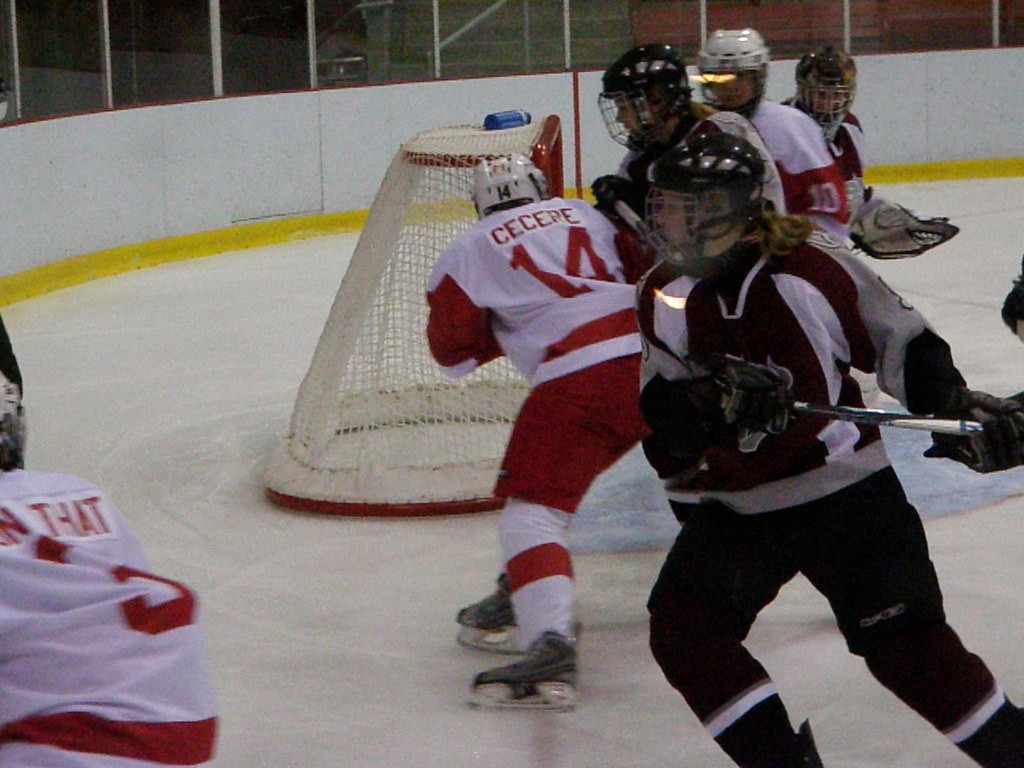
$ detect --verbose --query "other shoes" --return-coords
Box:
[795,718,824,768]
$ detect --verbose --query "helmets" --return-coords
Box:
[600,43,696,151]
[470,153,550,220]
[794,46,858,121]
[647,131,768,278]
[695,28,771,114]
[0,313,26,472]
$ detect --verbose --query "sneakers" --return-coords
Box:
[465,622,583,708]
[455,573,523,653]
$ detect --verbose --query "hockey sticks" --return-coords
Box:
[717,389,1024,438]
[590,176,665,253]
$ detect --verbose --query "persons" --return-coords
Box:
[638,133,1024,767]
[427,28,958,700]
[0,317,218,768]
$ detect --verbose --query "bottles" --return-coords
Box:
[484,109,531,129]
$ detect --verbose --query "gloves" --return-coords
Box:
[591,175,637,222]
[688,99,718,119]
[922,385,1024,474]
[705,352,794,435]
